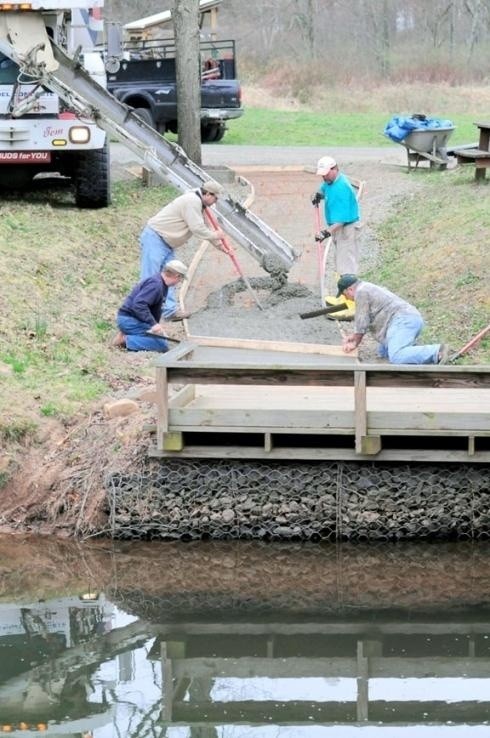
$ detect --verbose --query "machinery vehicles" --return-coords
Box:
[0,4,115,209]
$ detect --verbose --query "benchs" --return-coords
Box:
[453,148,490,181]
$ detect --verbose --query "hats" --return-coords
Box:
[316,157,336,176]
[336,276,356,297]
[167,260,188,282]
[203,182,220,198]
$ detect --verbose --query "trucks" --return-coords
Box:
[109,34,241,143]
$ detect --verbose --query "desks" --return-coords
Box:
[123,0,223,48]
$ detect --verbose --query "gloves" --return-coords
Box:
[315,231,330,242]
[312,192,321,204]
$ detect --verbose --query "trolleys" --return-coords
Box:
[389,107,458,176]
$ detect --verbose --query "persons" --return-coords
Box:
[336,273,450,365]
[309,154,361,276]
[112,259,190,353]
[138,179,234,322]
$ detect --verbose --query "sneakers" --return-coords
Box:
[439,344,448,364]
[111,332,124,348]
